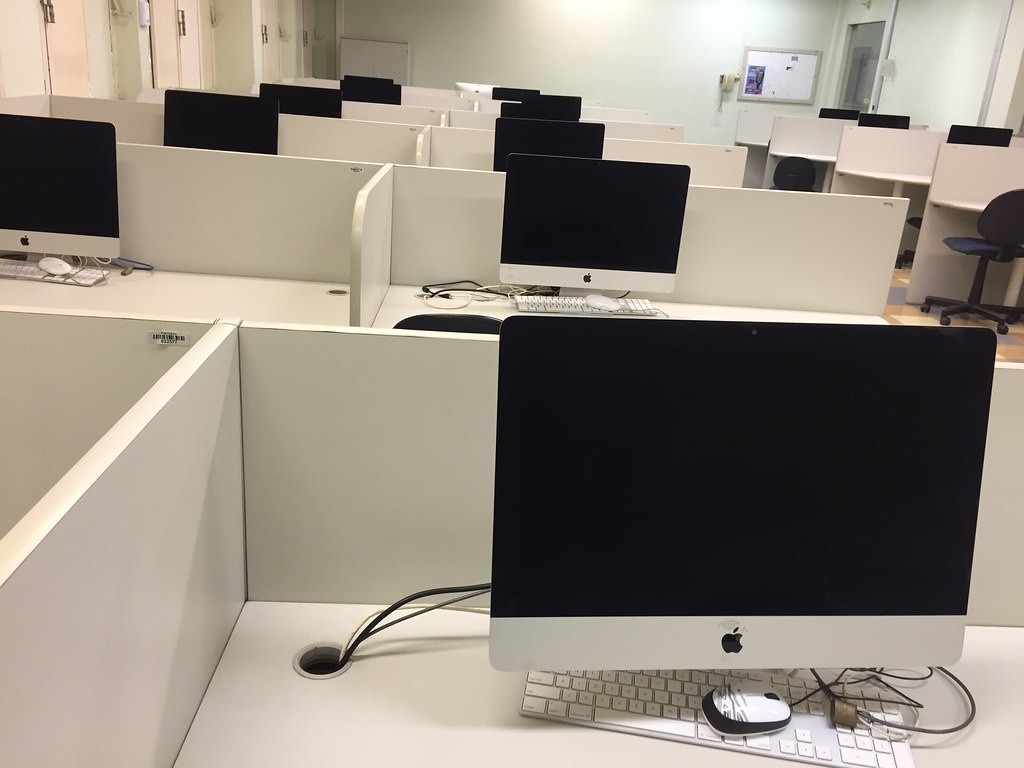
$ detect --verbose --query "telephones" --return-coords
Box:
[721,74,735,92]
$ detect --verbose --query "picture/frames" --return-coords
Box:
[738,45,821,104]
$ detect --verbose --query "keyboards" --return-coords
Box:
[0,258,109,287]
[518,671,917,768]
[514,295,657,317]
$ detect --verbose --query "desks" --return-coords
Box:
[0,140,1024,768]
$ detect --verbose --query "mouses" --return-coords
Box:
[700,681,792,738]
[38,256,72,275]
[585,294,621,311]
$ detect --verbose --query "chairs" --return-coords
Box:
[766,156,816,193]
[920,188,1023,335]
[895,216,922,270]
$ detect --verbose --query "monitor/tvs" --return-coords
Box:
[490,315,998,672]
[0,113,121,265]
[819,107,860,121]
[163,74,401,155]
[947,125,1014,148]
[455,82,692,293]
[858,113,910,130]
